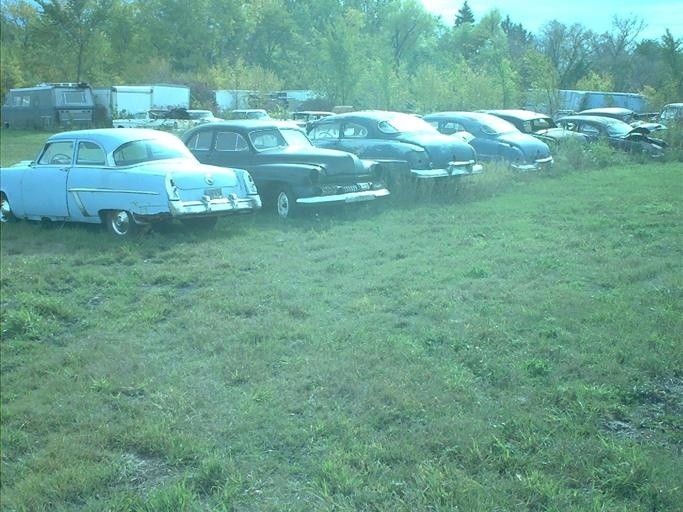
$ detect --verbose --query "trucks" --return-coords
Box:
[211,88,330,114]
[2,82,192,131]
